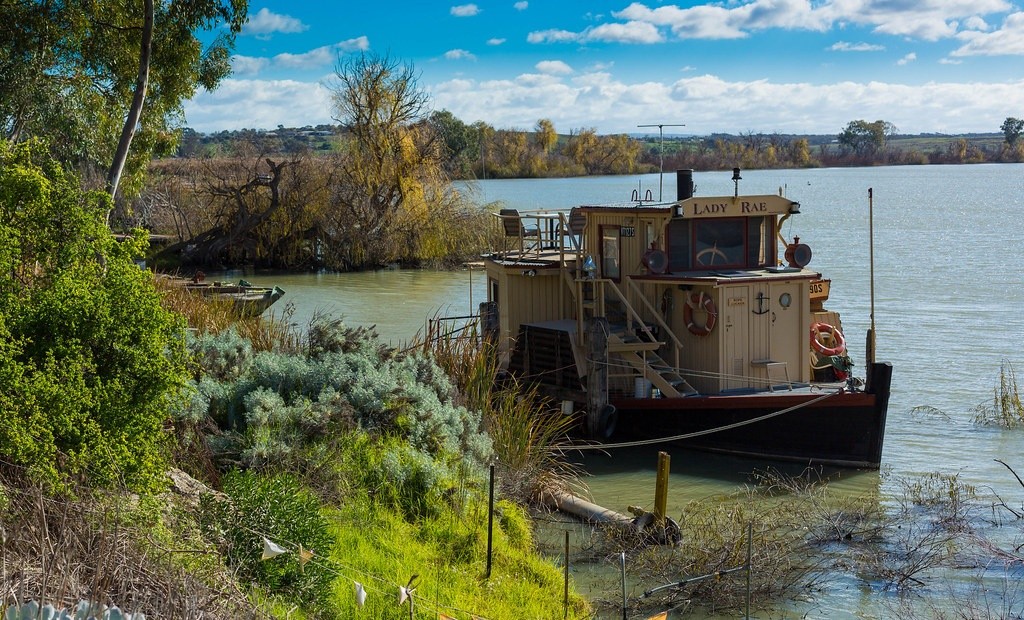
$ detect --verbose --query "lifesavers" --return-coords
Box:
[811,324,845,355]
[683,291,716,338]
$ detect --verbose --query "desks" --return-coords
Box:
[519,214,570,250]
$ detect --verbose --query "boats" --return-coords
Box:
[423,125,895,475]
[167,271,257,294]
[201,285,286,321]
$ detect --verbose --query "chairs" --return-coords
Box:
[555,206,587,253]
[500,209,544,253]
